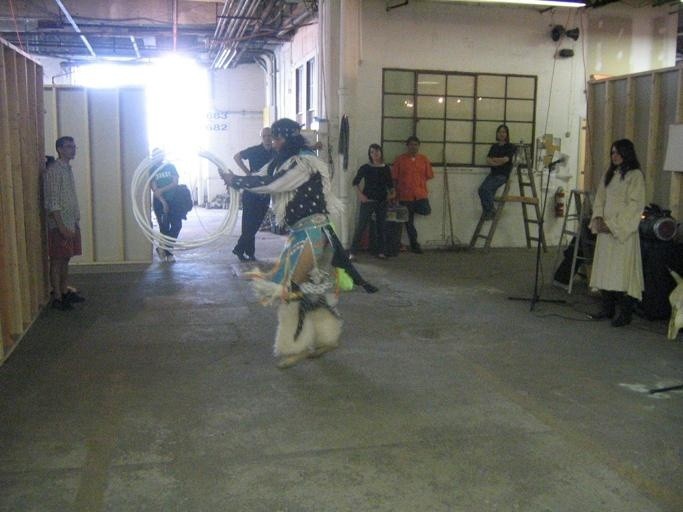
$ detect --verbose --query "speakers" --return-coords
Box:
[480,200,496,220]
[591,294,615,319]
[613,298,633,327]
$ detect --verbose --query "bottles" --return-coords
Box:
[272,126,305,146]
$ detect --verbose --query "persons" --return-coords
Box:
[389,136,435,252]
[588,139,645,327]
[149,146,182,264]
[40,134,85,313]
[477,124,517,220]
[229,127,323,264]
[349,142,396,262]
[216,118,339,369]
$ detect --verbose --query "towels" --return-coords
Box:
[369,204,408,257]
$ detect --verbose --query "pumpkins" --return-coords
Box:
[551,189,596,295]
[465,141,548,253]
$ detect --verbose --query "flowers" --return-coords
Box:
[548,157,565,166]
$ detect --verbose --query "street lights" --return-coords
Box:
[174,184,193,213]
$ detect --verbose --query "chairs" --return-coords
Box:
[361,281,377,293]
[233,245,255,261]
[54,299,72,311]
[63,290,85,303]
[156,247,175,262]
